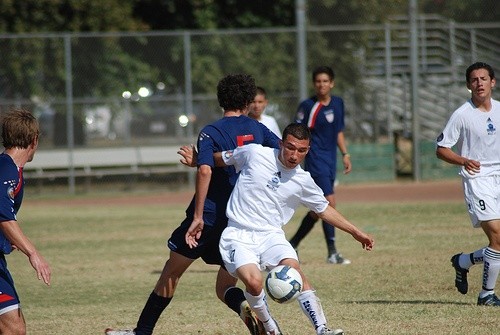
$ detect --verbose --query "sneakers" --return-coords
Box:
[105,326,135,335]
[477,292,500,306]
[451,252,470,294]
[239,301,266,335]
[318,326,343,335]
[328,254,351,264]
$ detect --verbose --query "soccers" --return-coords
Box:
[265,265,303,305]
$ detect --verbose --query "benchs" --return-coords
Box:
[22,145,196,190]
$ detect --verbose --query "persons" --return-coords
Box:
[434,61,500,306]
[104,74,283,335]
[248,86,282,139]
[0,109,52,335]
[289,66,354,264]
[177,122,374,335]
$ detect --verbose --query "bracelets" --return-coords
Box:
[343,152,350,157]
